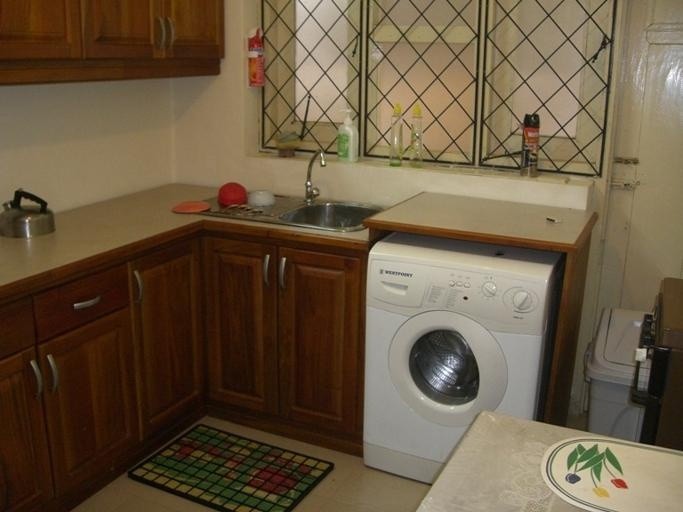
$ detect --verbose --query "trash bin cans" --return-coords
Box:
[583,306,656,443]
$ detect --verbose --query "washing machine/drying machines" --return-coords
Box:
[361,232,565,485]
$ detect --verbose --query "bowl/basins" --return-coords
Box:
[248,190,275,208]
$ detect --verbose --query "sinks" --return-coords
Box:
[281,199,385,232]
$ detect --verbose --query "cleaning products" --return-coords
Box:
[337,108,359,161]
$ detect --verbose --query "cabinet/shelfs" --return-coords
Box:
[1,0,80,85]
[203,232,366,459]
[127,229,203,458]
[1,263,143,511]
[80,0,225,82]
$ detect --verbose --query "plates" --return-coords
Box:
[171,199,211,214]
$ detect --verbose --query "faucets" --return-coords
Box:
[304,148,327,202]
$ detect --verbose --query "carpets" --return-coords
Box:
[129,426,335,511]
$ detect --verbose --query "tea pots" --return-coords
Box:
[0,189,55,238]
